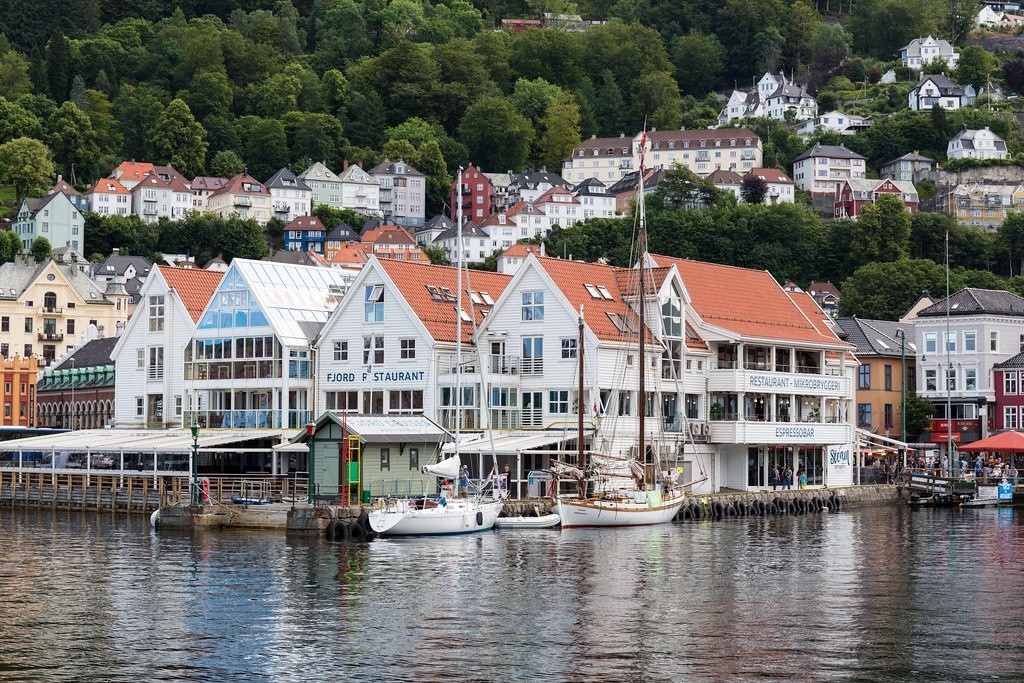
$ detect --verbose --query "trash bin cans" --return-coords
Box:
[363,490,370,503]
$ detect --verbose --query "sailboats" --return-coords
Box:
[365,167,503,536]
[552,166,688,528]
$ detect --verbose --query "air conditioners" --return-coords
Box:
[689,423,701,436]
[701,424,711,436]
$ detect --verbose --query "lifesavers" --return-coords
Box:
[773,497,786,512]
[824,499,834,510]
[793,497,806,511]
[498,512,508,517]
[326,522,347,540]
[681,507,693,520]
[522,510,538,517]
[477,511,482,524]
[812,496,824,510]
[749,505,757,515]
[734,500,747,516]
[346,523,364,542]
[754,499,766,514]
[712,502,725,516]
[787,500,796,512]
[357,516,377,539]
[689,503,702,518]
[830,495,840,509]
[806,500,815,512]
[725,504,737,516]
[767,503,777,512]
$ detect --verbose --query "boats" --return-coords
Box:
[493,513,560,530]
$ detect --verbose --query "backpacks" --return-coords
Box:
[771,469,777,478]
[783,469,789,478]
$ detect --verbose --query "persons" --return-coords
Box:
[500,464,511,499]
[439,464,469,499]
[772,450,1024,492]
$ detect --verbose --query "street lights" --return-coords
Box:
[305,420,317,504]
[190,418,202,505]
